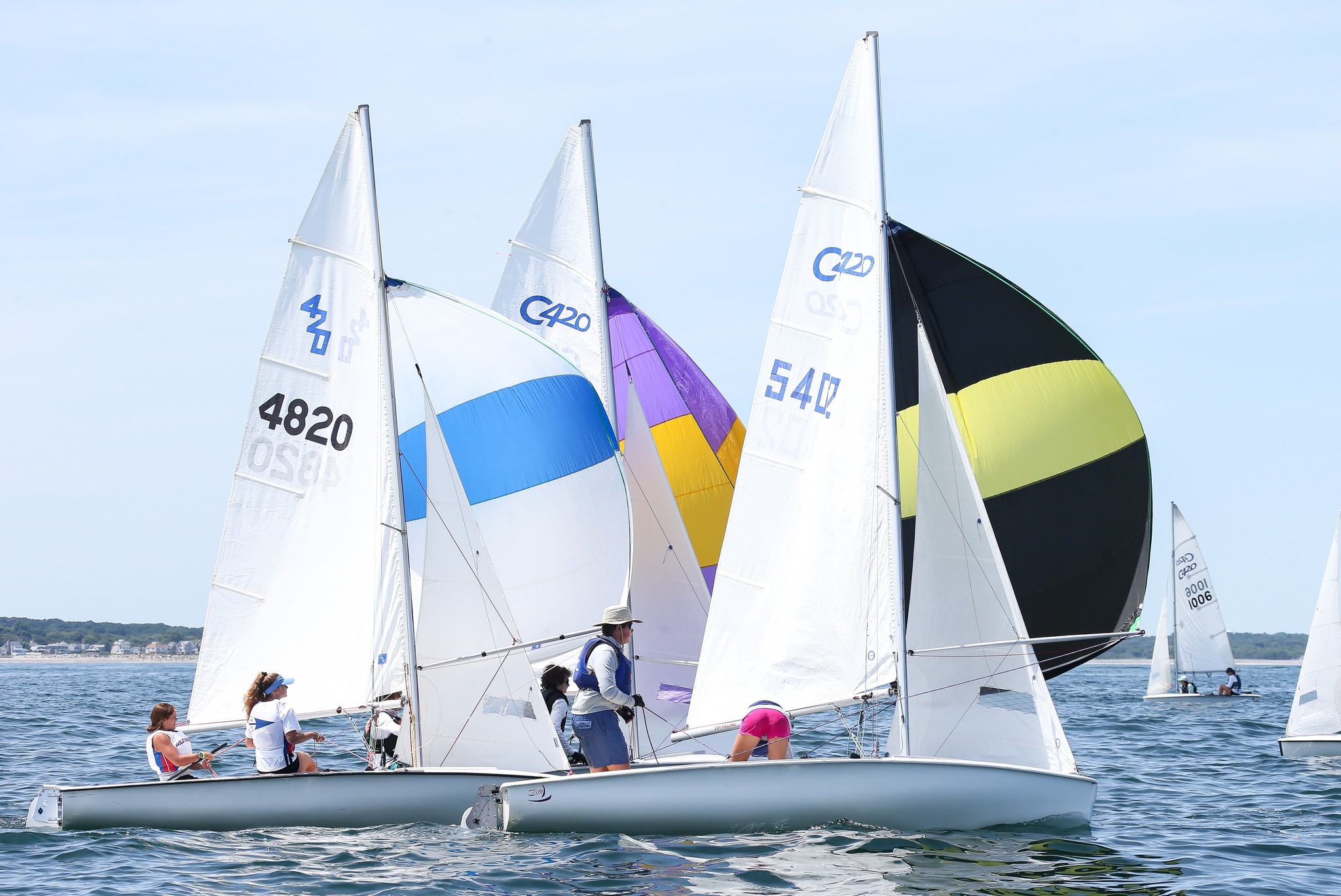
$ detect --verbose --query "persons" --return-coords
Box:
[571,604,646,773]
[145,703,214,782]
[1219,668,1241,696]
[368,691,411,771]
[729,700,794,762]
[1179,675,1196,693]
[243,671,325,774]
[541,665,590,767]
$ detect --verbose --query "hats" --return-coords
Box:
[1177,675,1188,681]
[591,605,644,626]
[1226,668,1233,672]
[263,675,295,695]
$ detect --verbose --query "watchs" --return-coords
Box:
[197,752,203,760]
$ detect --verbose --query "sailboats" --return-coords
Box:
[19,101,636,839]
[465,34,1155,834]
[1277,520,1341,756]
[1137,503,1259,702]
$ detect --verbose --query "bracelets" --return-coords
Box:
[199,751,205,759]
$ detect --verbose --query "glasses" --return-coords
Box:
[751,754,768,757]
[564,679,569,685]
[1226,671,1231,673]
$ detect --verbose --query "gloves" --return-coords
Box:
[567,751,589,766]
